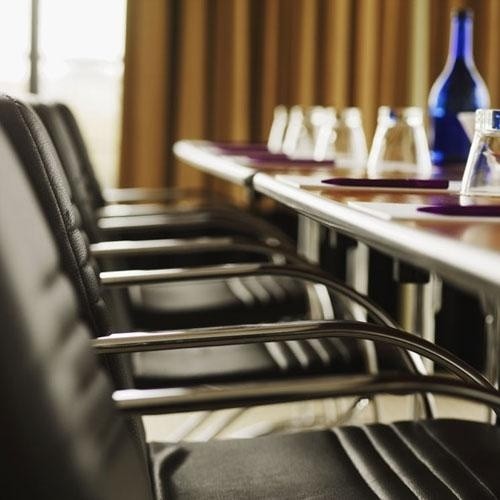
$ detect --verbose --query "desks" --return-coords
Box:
[171,136,499,390]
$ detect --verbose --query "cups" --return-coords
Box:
[457,109,500,209]
[367,106,431,182]
[265,103,366,176]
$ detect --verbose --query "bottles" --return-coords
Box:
[428,8,490,181]
